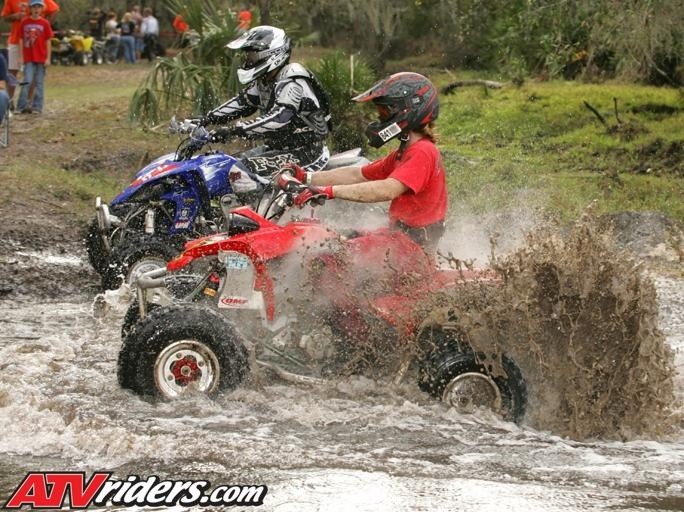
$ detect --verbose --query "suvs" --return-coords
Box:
[112,157,529,427]
[81,115,385,293]
[50,34,106,66]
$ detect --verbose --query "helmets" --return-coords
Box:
[352,71,440,148]
[223,25,292,86]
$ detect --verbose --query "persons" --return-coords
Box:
[236,4,252,38]
[51,5,158,64]
[0,54,16,126]
[13,0,53,116]
[277,70,448,381]
[172,4,192,50]
[192,25,331,209]
[1,0,59,114]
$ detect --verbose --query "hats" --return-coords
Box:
[28,0,45,9]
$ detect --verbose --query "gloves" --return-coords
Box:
[280,161,307,184]
[189,116,212,128]
[292,180,335,210]
[206,123,236,144]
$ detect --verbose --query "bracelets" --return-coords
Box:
[305,170,312,184]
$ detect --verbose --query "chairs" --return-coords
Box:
[45,32,118,65]
